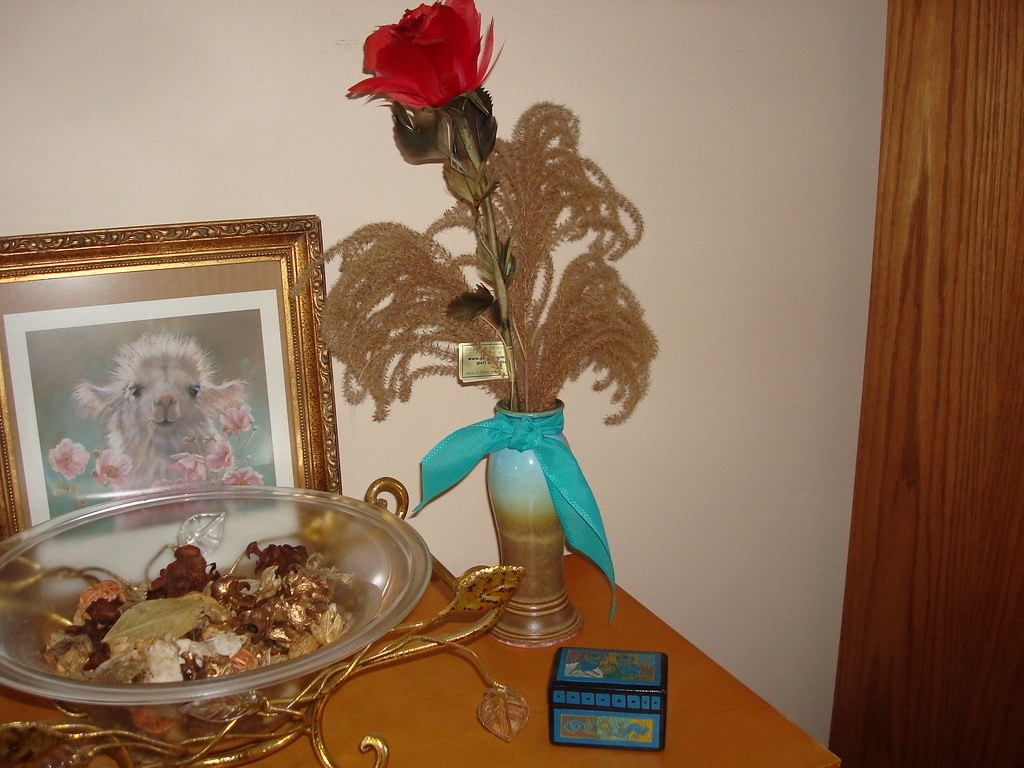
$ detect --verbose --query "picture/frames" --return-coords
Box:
[0,214,344,559]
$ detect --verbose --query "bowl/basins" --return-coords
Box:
[2,484,432,757]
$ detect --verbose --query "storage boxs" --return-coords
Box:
[550,646,669,750]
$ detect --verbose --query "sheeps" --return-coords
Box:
[70,330,251,532]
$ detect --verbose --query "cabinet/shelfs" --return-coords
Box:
[0,551,842,768]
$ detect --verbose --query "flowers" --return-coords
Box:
[283,1,659,426]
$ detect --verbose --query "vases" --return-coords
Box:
[485,399,582,650]
